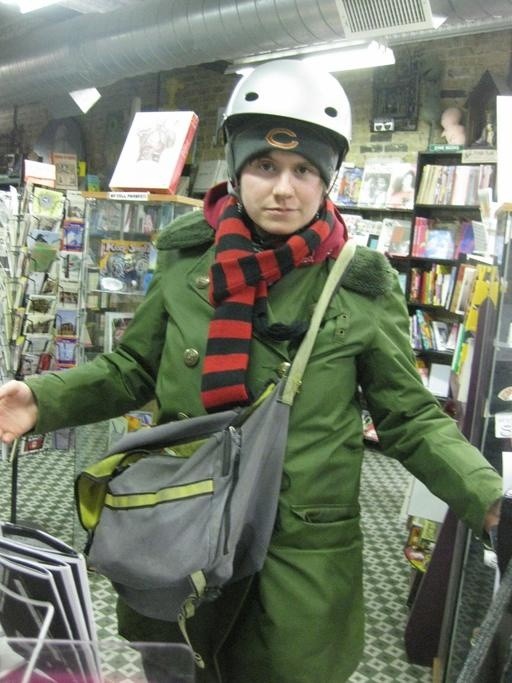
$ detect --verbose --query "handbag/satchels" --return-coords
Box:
[74,375,291,624]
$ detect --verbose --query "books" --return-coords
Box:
[331,157,416,295]
[0,522,97,682]
[408,165,502,403]
[1,188,170,457]
[108,109,200,194]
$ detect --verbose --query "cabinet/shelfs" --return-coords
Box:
[400,149,499,417]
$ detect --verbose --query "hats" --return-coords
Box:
[224,116,340,189]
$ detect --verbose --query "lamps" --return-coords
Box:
[222,37,397,83]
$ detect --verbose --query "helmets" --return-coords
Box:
[220,59,352,158]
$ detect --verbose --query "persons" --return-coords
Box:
[0,59,506,683]
